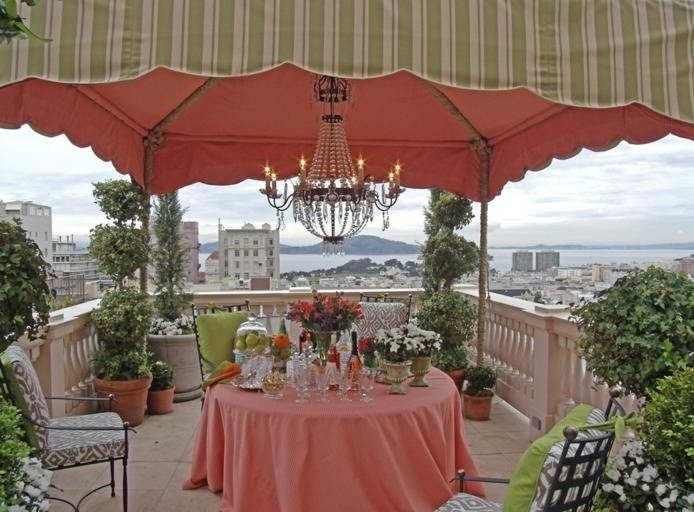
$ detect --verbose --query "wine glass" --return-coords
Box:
[292,363,377,404]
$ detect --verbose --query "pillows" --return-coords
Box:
[0,345,50,452]
[355,300,408,343]
[194,309,249,370]
[497,398,611,512]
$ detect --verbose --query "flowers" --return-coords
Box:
[147,313,198,335]
[276,285,366,329]
[371,325,415,363]
[397,320,444,358]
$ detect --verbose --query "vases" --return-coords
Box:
[308,326,336,364]
[405,353,432,387]
[380,358,411,396]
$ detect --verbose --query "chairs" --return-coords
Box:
[357,289,412,352]
[420,385,629,510]
[188,297,255,409]
[0,337,136,512]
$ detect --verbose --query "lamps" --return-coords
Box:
[258,72,408,256]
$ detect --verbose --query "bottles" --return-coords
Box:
[326,333,341,370]
[347,331,362,391]
[272,318,291,374]
[339,339,352,364]
[298,329,311,355]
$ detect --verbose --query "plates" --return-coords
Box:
[229,377,264,394]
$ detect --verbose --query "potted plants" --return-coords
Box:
[143,183,206,405]
[457,361,497,424]
[82,175,159,427]
[145,359,180,416]
[409,186,472,393]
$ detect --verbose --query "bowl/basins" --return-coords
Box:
[261,377,286,400]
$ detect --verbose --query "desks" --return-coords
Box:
[181,367,489,511]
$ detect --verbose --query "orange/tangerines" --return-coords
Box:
[234,331,291,360]
